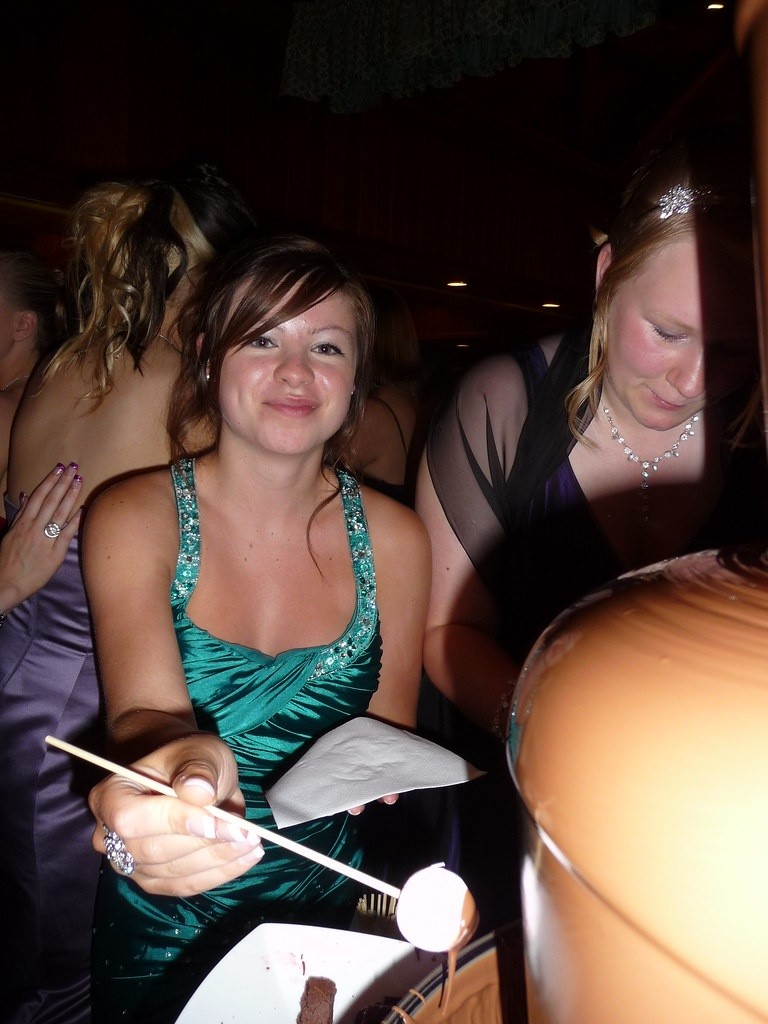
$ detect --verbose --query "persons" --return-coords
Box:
[1,249,81,540]
[77,237,431,1024]
[328,274,422,512]
[0,462,85,613]
[410,156,766,976]
[0,157,264,1024]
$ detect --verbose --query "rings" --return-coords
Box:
[103,827,135,875]
[44,522,61,538]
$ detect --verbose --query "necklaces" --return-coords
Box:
[155,332,174,347]
[0,375,30,392]
[599,399,698,529]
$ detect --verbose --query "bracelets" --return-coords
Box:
[492,674,519,741]
